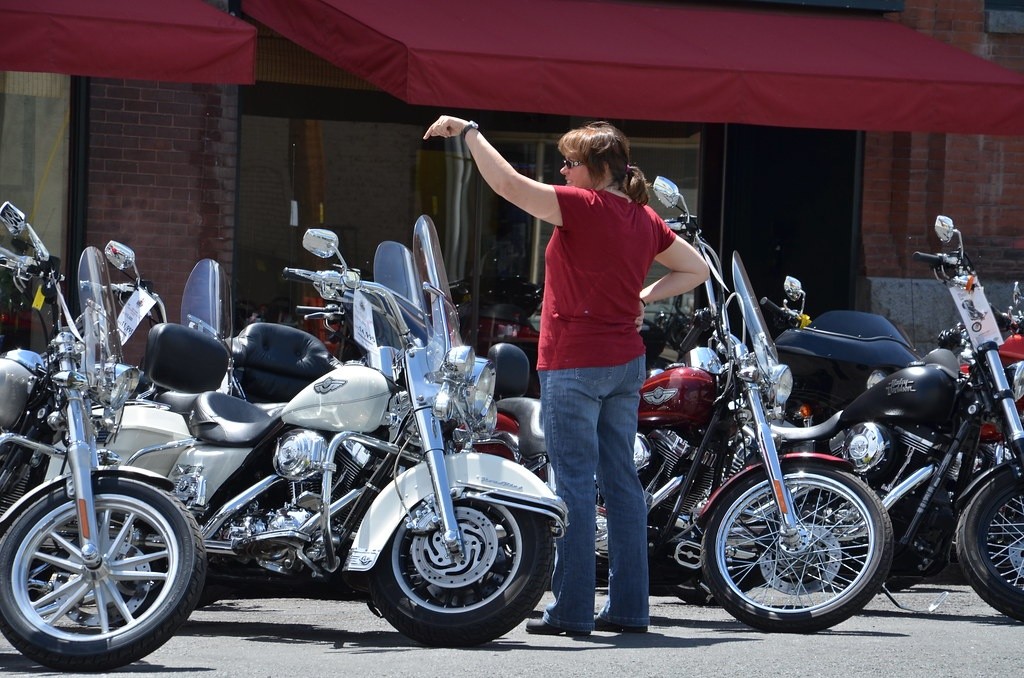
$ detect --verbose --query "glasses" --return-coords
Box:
[563,159,588,168]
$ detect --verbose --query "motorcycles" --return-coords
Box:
[0,177,1023,673]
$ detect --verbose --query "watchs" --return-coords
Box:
[462,120,478,138]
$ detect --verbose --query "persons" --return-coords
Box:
[423,115,710,638]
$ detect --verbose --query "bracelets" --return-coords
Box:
[640,297,646,308]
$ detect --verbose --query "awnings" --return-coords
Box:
[240,0,1024,135]
[0,1,258,85]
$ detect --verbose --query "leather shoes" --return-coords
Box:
[525,619,591,636]
[594,616,648,633]
